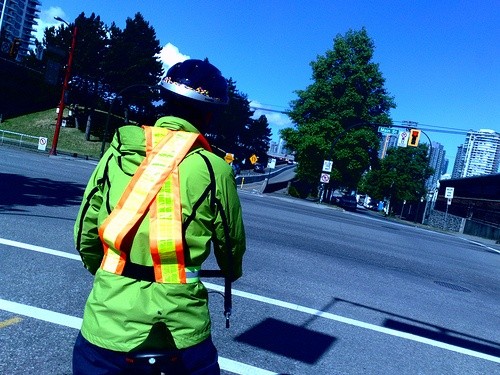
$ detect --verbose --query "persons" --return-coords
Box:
[72,58,246,375]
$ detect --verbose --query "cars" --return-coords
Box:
[254,164,264,173]
[331,189,358,212]
[356,194,387,213]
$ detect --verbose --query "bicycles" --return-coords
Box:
[125,267,232,375]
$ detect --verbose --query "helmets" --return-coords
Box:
[161,59,229,108]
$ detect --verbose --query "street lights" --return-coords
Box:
[47,15,81,156]
[408,128,421,147]
